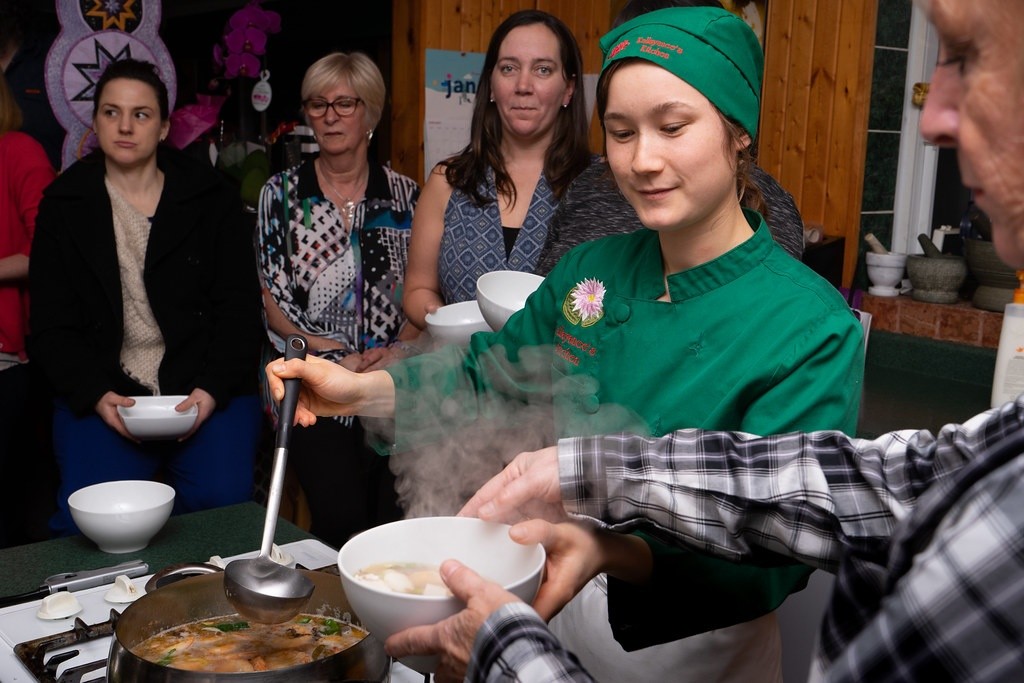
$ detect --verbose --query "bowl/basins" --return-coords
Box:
[907,251,966,303]
[866,252,905,297]
[66,481,177,557]
[116,393,194,438]
[966,237,1022,314]
[426,299,494,348]
[335,514,546,675]
[477,269,548,333]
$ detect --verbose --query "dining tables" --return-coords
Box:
[1,502,507,683]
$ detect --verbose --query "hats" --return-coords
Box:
[598,6,764,144]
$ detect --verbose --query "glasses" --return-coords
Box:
[303,96,364,118]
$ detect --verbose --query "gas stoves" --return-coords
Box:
[2,537,435,682]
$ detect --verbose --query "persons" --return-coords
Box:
[261,6,865,683]
[20,59,268,538]
[378,1,1024,683]
[0,73,62,463]
[252,49,430,553]
[403,8,607,359]
[212,92,281,212]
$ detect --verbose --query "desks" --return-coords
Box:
[806,285,1023,412]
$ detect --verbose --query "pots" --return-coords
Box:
[102,565,392,683]
